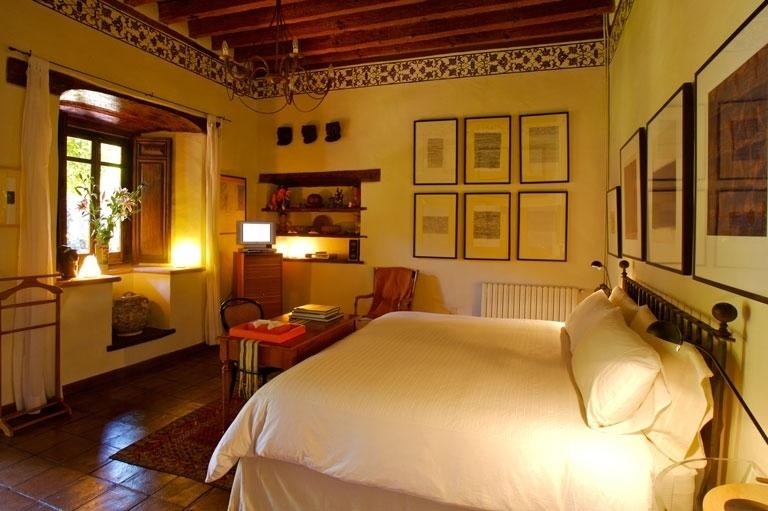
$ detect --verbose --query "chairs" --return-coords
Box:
[220,297,284,400]
[349,266,419,331]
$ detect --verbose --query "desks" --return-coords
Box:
[216,313,361,437]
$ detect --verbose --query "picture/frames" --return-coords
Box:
[412,111,569,263]
[217,173,247,235]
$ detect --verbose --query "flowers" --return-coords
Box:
[73,172,145,261]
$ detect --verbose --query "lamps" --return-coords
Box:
[217,0,335,116]
[590,259,613,296]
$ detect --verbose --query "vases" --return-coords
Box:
[93,240,110,273]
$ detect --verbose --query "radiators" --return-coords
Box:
[478,281,581,323]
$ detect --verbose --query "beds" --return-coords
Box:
[205,259,738,511]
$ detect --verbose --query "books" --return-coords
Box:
[289,304,344,323]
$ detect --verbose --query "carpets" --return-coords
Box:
[108,384,264,491]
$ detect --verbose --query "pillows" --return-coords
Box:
[564,285,697,443]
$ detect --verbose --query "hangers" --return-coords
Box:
[0,278,64,300]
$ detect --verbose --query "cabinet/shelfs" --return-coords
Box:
[231,250,283,319]
[260,182,368,265]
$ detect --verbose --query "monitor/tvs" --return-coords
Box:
[236,220,275,250]
